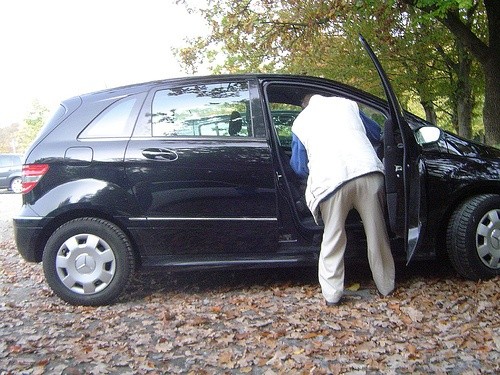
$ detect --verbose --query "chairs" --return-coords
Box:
[229,111,243,136]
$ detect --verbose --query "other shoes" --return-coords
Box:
[326,298,340,307]
[378,290,393,299]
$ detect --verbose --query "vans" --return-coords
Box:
[12,31,500,306]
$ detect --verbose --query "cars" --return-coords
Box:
[0,154,24,194]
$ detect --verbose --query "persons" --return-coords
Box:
[288,93,395,307]
